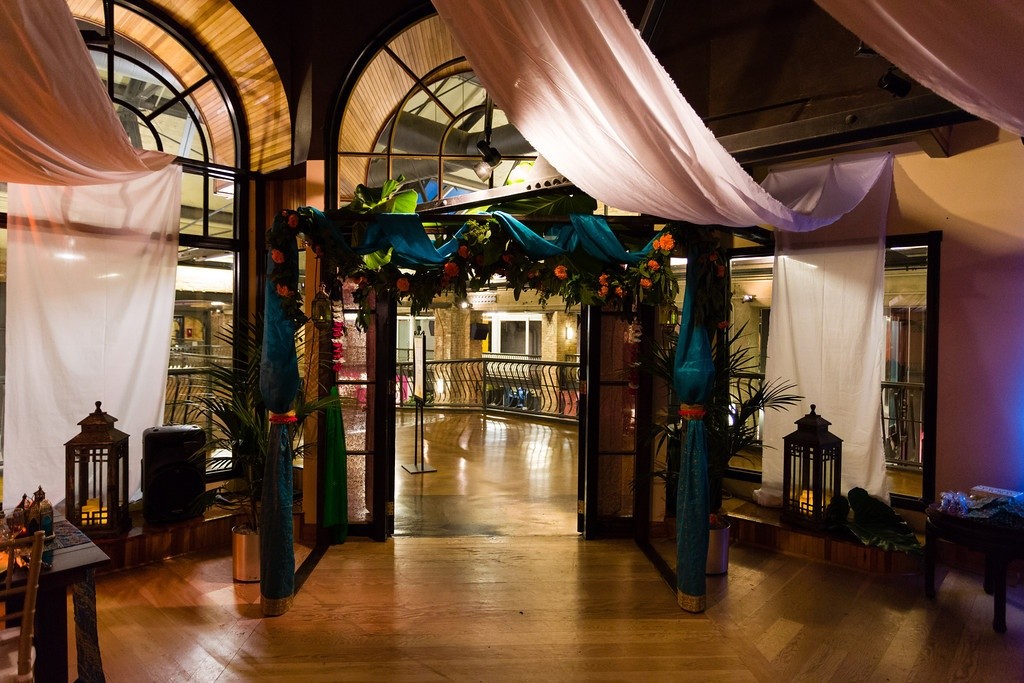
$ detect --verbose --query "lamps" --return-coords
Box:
[780,401,847,532]
[471,137,506,184]
[873,65,916,101]
[62,398,135,541]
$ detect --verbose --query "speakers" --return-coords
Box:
[472,322,489,340]
[140,423,206,527]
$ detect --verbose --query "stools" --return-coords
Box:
[921,515,1024,632]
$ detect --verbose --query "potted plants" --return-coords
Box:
[620,316,810,579]
[169,310,360,584]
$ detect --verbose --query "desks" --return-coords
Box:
[0,507,112,683]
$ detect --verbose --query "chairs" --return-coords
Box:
[0,529,47,676]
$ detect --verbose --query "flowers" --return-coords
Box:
[250,204,742,333]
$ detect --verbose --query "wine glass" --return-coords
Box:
[0,508,25,571]
